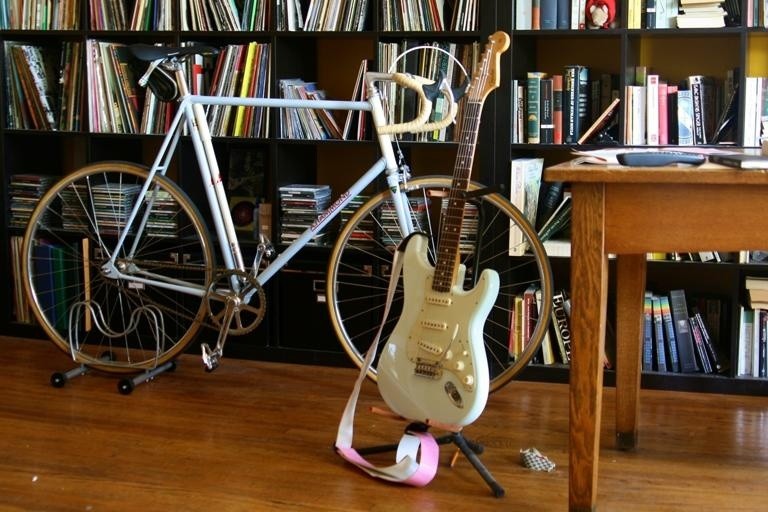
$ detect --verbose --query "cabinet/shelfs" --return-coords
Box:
[0,0,768,396]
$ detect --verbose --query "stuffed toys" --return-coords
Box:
[586,1,619,30]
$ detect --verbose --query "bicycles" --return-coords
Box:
[18,43,553,395]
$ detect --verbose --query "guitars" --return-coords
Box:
[377,29,511,427]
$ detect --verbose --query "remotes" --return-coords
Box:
[615,150,705,167]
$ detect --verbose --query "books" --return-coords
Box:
[441,197,479,255]
[514,64,621,144]
[509,157,618,258]
[570,147,767,169]
[627,2,742,28]
[144,190,181,238]
[745,77,767,148]
[2,2,267,31]
[278,183,332,246]
[11,235,98,332]
[627,66,739,145]
[276,1,367,31]
[340,193,376,248]
[59,183,91,231]
[279,59,370,141]
[376,41,480,142]
[379,196,431,251]
[508,284,615,370]
[92,183,142,234]
[3,39,271,139]
[746,2,766,28]
[643,250,766,379]
[383,3,476,31]
[513,2,586,31]
[8,174,51,229]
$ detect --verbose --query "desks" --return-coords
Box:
[542,156,768,512]
